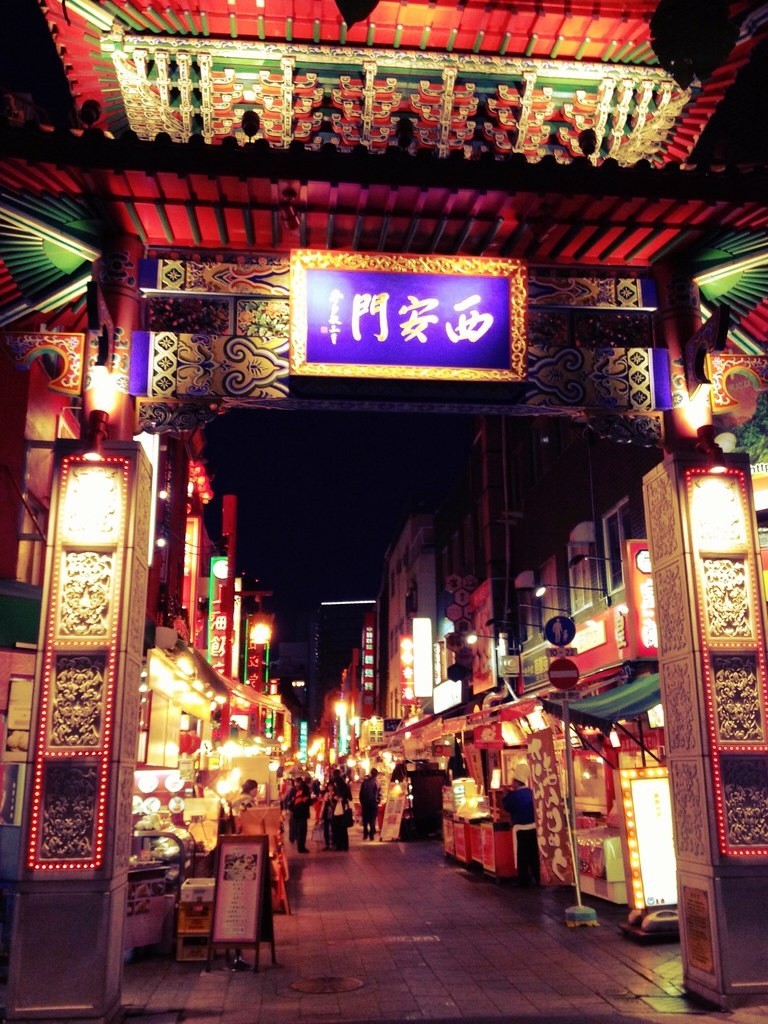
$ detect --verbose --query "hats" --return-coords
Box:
[513,763,529,785]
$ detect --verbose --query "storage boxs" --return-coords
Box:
[175,932,212,963]
[180,877,216,903]
[176,900,214,933]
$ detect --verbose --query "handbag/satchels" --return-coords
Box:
[340,798,354,829]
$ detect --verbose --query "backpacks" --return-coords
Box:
[363,777,378,803]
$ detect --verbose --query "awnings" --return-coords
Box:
[536,671,666,771]
[384,693,553,759]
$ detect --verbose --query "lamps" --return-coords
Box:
[485,618,545,636]
[534,585,611,606]
[464,633,524,653]
[567,553,624,569]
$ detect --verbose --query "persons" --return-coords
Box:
[280,766,382,855]
[499,763,547,890]
[229,780,261,813]
